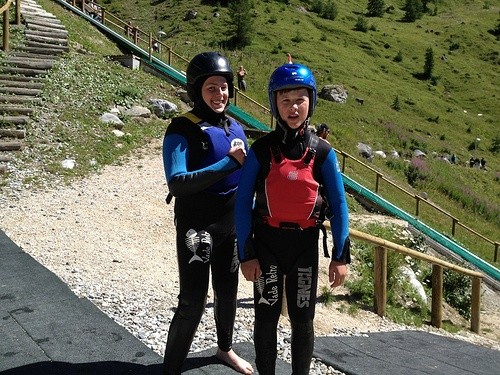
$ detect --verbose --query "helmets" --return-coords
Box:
[186,51,235,103]
[268,63,317,120]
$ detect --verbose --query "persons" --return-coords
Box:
[234,63,351,375]
[237,65,247,91]
[162,51,253,374]
[152,36,159,53]
[125,20,140,43]
